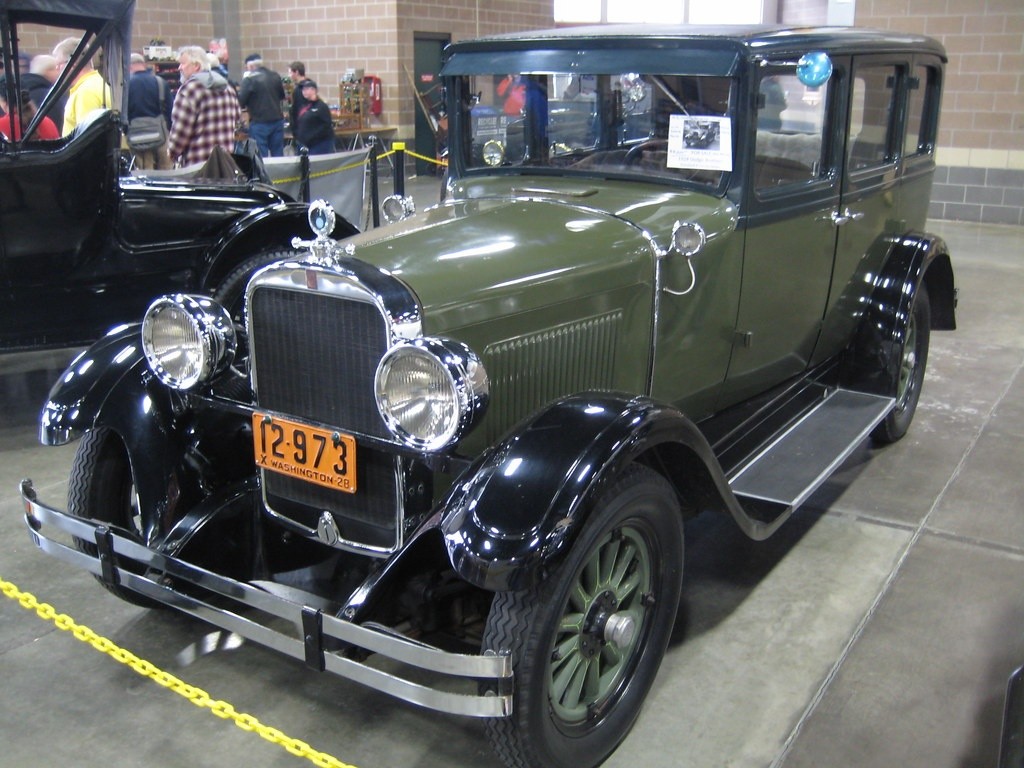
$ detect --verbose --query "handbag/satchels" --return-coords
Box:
[126,114,169,151]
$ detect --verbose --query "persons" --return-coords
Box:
[122,53,173,171]
[52,38,129,150]
[0,49,69,141]
[206,51,239,97]
[287,62,334,155]
[236,54,285,157]
[166,46,241,167]
[498,74,527,165]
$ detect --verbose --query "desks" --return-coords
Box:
[337,125,398,172]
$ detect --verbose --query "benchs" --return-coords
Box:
[571,149,812,193]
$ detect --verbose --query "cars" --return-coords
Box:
[0,0,361,410]
[18,22,959,768]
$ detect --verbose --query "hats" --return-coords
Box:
[299,79,317,87]
[245,53,260,63]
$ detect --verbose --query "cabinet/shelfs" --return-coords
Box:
[332,84,371,128]
